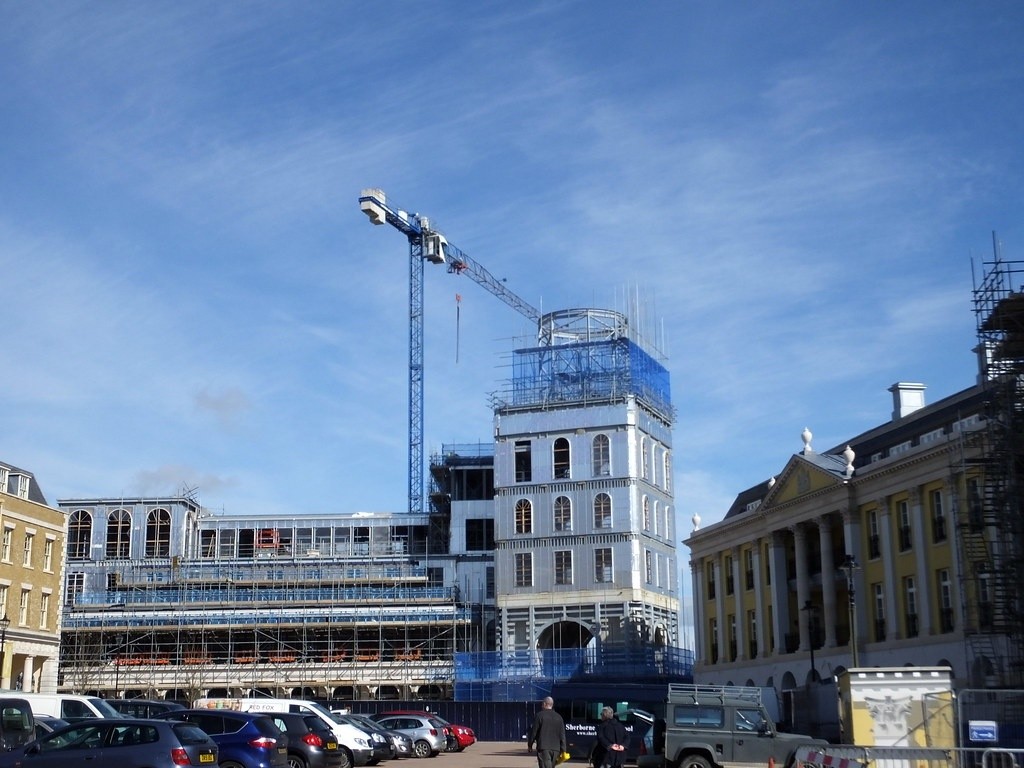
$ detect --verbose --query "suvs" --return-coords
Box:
[662,683,830,768]
[612,707,760,768]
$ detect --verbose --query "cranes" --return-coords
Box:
[357,187,565,516]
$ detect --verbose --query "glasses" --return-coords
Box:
[600,713,604,715]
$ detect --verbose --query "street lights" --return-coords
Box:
[837,553,861,669]
[803,600,818,682]
[0,613,11,688]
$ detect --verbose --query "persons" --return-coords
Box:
[592,707,631,768]
[527,696,566,768]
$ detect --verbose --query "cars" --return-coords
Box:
[0,688,475,768]
